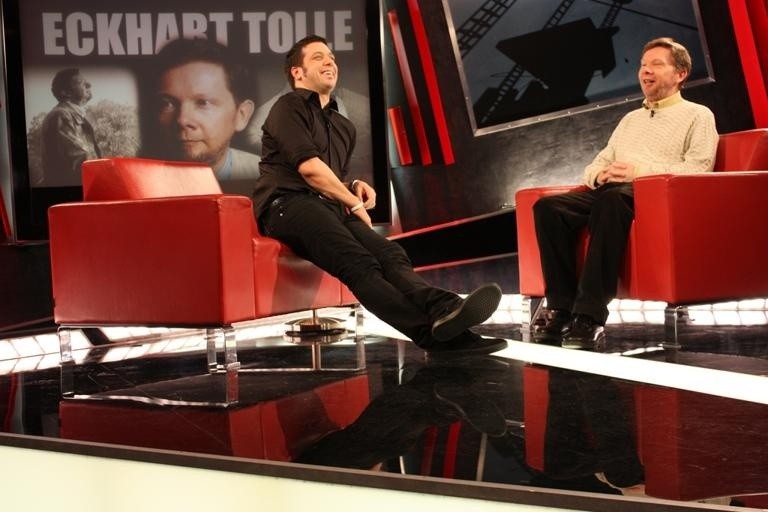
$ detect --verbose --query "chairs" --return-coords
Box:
[515,127,768,348]
[47,157,365,409]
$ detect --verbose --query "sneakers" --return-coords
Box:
[431,282,502,342]
[425,332,507,356]
[425,354,508,437]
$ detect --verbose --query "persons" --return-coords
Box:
[530,37,721,341]
[248,82,348,144]
[544,366,645,494]
[291,358,509,471]
[252,36,507,360]
[39,68,103,187]
[138,37,264,181]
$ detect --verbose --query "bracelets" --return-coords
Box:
[350,203,363,213]
[350,179,357,191]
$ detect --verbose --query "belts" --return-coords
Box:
[268,192,292,210]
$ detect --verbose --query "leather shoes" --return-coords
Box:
[533,308,568,341]
[562,313,605,346]
